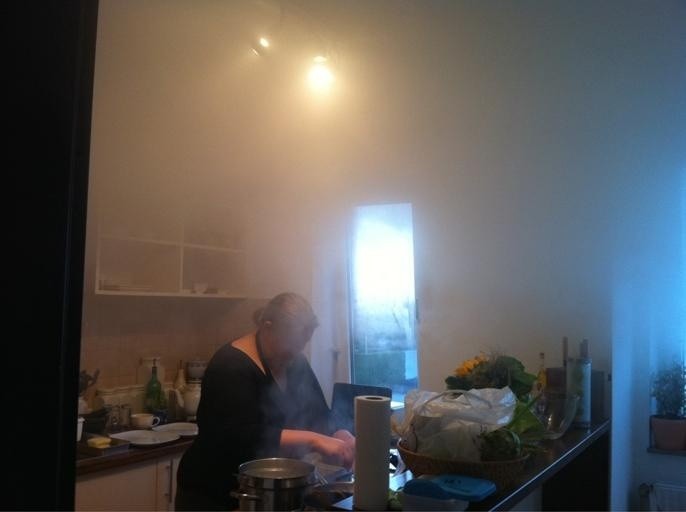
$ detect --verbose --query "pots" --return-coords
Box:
[231,458,317,510]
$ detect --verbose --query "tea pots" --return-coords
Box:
[171,383,201,420]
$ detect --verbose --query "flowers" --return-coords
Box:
[444,350,536,399]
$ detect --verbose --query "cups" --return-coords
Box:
[187,361,209,381]
[77,417,84,442]
[193,281,209,295]
[131,413,160,428]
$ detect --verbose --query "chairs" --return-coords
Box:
[329,380,395,442]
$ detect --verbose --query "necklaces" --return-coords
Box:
[269,366,287,392]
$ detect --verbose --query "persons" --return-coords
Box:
[173,291,357,512]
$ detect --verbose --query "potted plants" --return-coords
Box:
[645,360,685,451]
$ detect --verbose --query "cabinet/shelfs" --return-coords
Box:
[75,455,185,511]
[95,190,251,300]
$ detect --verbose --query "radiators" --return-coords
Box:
[640,480,686,512]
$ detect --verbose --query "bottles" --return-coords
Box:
[535,352,548,391]
[93,383,148,427]
[174,359,186,389]
[565,356,593,429]
[145,358,161,411]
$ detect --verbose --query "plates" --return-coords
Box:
[108,422,200,446]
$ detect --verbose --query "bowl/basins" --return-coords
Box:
[526,389,579,441]
[79,414,109,434]
